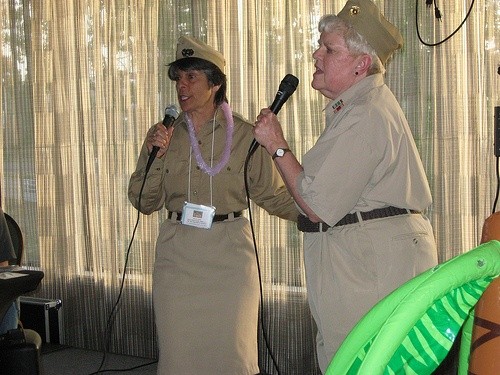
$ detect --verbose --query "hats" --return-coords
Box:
[337,0,403,65]
[176,35,226,74]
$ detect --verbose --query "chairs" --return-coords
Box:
[0,213,23,266]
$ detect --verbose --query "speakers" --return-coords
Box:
[0,343,39,375]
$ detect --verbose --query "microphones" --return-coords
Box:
[146,104,181,173]
[248,74,299,157]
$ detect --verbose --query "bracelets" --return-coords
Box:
[271,147,292,161]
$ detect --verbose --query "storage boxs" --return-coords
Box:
[20,296,62,345]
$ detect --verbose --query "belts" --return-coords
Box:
[298,206,422,232]
[168,211,242,222]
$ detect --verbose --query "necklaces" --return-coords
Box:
[185,102,234,177]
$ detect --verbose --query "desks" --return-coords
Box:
[0,266,44,326]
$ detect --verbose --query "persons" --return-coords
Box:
[252,0,439,374]
[129,36,301,375]
